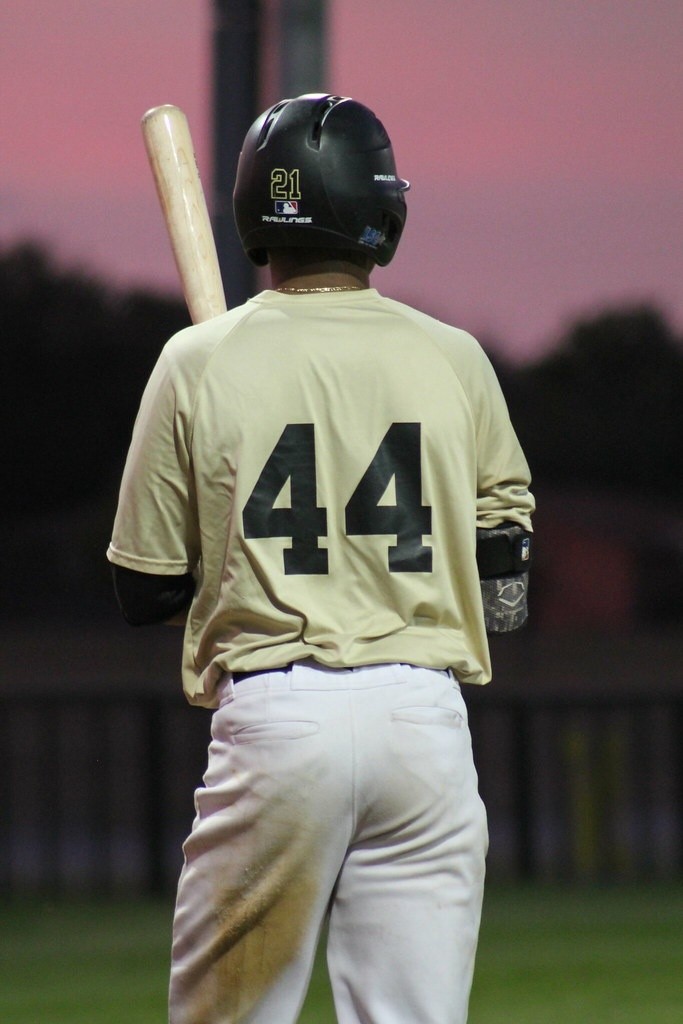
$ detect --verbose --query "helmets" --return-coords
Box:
[232,92,411,267]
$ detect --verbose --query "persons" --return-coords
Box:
[108,91,536,1024]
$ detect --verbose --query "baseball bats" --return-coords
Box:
[143,99,228,324]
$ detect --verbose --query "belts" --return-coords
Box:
[231,662,292,682]
